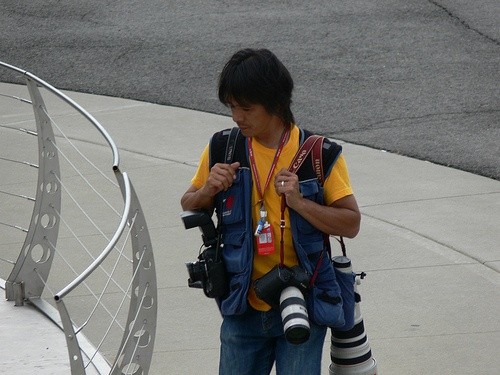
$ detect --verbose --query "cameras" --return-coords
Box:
[180,208,228,298]
[254,263,311,344]
[329,256,377,375]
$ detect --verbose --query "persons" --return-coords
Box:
[180,47,362,375]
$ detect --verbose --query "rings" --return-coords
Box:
[281,181,285,187]
